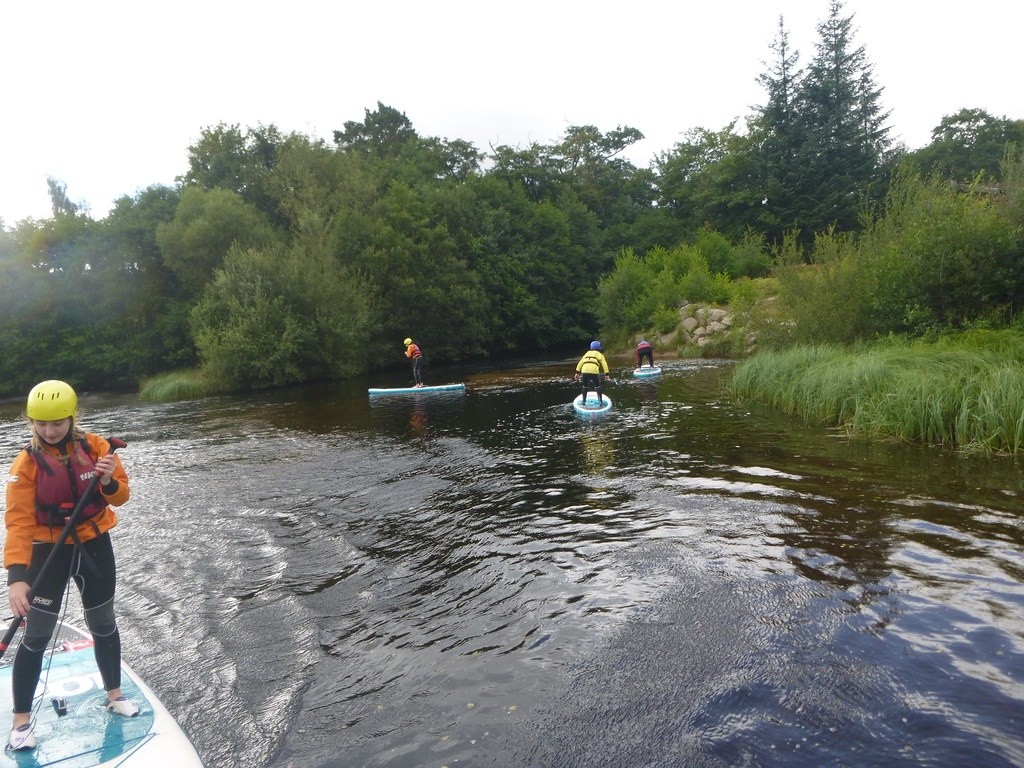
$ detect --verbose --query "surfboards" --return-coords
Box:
[0,645,206,767]
[571,390,612,421]
[631,361,666,378]
[366,384,466,396]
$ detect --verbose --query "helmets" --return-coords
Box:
[26,380,78,430]
[404,338,413,345]
[590,341,601,350]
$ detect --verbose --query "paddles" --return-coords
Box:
[1,435,131,667]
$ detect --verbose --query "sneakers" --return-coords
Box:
[105,697,139,717]
[8,724,37,750]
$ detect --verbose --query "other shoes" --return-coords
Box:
[412,383,424,388]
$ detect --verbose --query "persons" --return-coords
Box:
[636,340,655,371]
[3,380,141,751]
[574,340,611,407]
[404,338,424,388]
